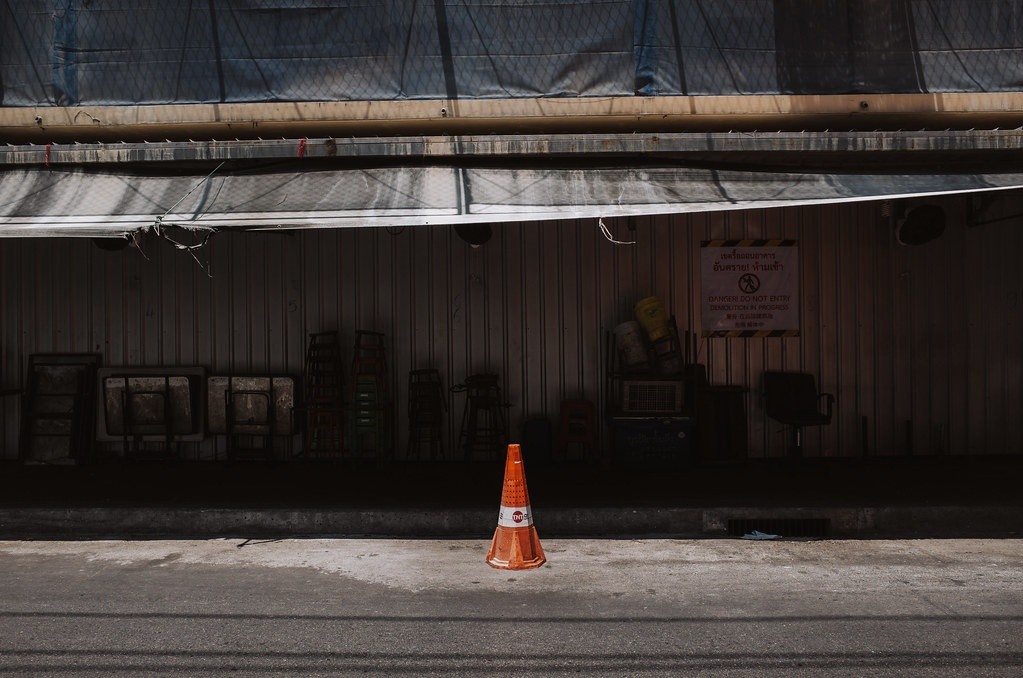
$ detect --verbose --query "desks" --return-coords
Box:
[603,422,695,473]
[23,352,302,471]
[603,319,697,418]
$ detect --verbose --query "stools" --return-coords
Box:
[522,418,554,466]
[647,318,681,383]
[559,399,596,470]
[352,328,393,465]
[454,374,512,463]
[405,367,447,464]
[300,330,346,466]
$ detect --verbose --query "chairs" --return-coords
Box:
[762,371,837,469]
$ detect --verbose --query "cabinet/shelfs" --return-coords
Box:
[692,385,750,468]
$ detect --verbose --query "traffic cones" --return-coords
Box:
[485,445,545,569]
